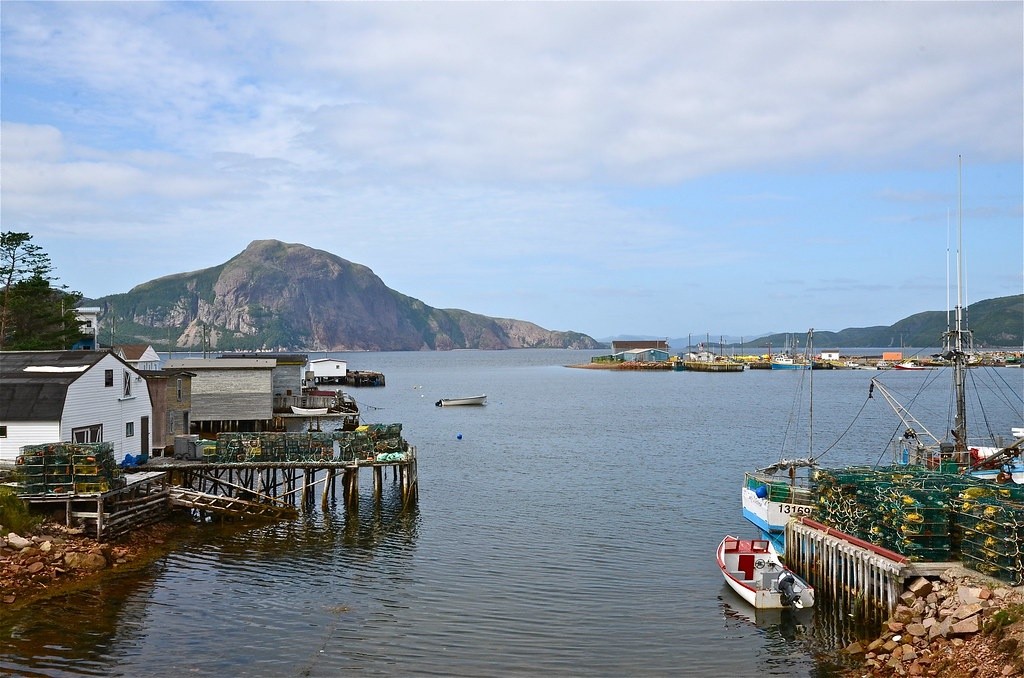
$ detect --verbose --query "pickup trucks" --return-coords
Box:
[845,361,859,367]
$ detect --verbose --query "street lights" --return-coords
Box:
[689,333,693,361]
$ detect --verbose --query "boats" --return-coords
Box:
[671,360,686,370]
[875,360,891,370]
[927,355,983,366]
[719,581,813,641]
[742,156,1024,553]
[1006,359,1023,369]
[894,360,926,370]
[436,394,489,406]
[770,352,813,370]
[291,405,327,415]
[715,535,815,612]
